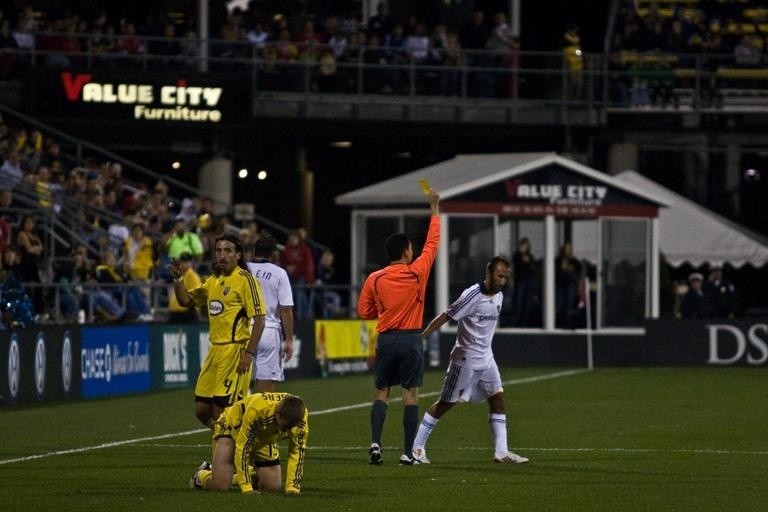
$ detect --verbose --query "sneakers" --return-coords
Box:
[401,443,430,464]
[494,453,529,463]
[369,443,383,464]
[190,461,210,489]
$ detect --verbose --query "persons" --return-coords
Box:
[412,257,531,466]
[510,238,737,326]
[243,234,294,395]
[2,123,339,322]
[0,0,768,96]
[189,391,308,496]
[167,234,267,430]
[355,195,441,465]
